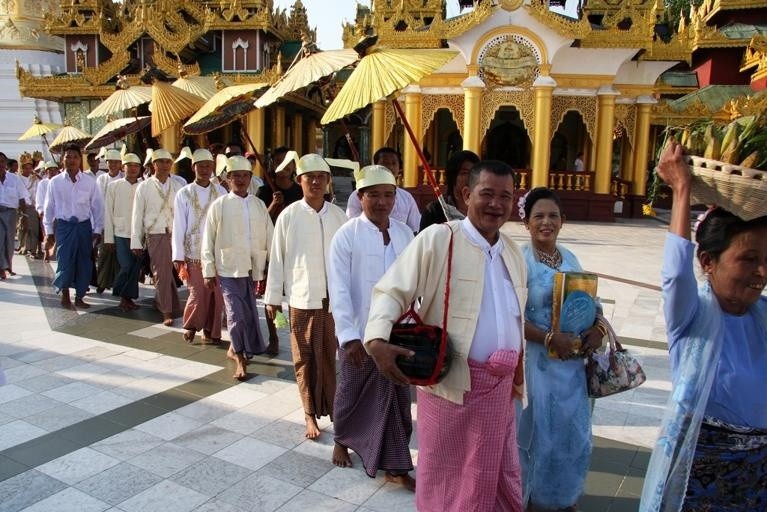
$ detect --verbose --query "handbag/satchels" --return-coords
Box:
[387,223,454,386]
[583,312,646,400]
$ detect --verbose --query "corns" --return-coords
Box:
[671,116,767,174]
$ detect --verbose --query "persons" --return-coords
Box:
[639,132,767,512]
[0,146,124,308]
[102,147,304,377]
[362,160,530,512]
[265,147,482,492]
[514,187,606,512]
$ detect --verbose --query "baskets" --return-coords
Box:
[682,152,767,220]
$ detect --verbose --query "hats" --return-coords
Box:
[33,152,44,162]
[19,153,33,166]
[45,160,58,170]
[356,164,397,191]
[296,153,331,177]
[105,149,121,162]
[191,148,214,164]
[226,155,252,173]
[152,149,173,163]
[121,152,141,166]
